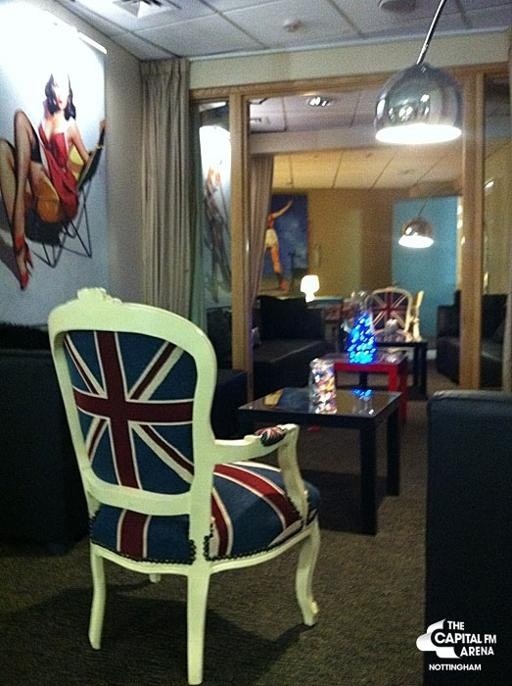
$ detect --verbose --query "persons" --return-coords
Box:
[263,198,295,290]
[0,69,91,293]
[203,164,232,303]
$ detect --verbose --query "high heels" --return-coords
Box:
[14,236,33,289]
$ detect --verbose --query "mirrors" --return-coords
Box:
[190,67,511,393]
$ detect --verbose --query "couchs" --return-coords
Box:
[422,390,512,684]
[0,348,250,558]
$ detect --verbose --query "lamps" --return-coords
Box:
[373,0,465,147]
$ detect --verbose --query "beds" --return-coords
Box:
[234,385,405,536]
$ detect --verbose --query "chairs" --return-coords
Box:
[22,123,107,269]
[47,286,322,686]
